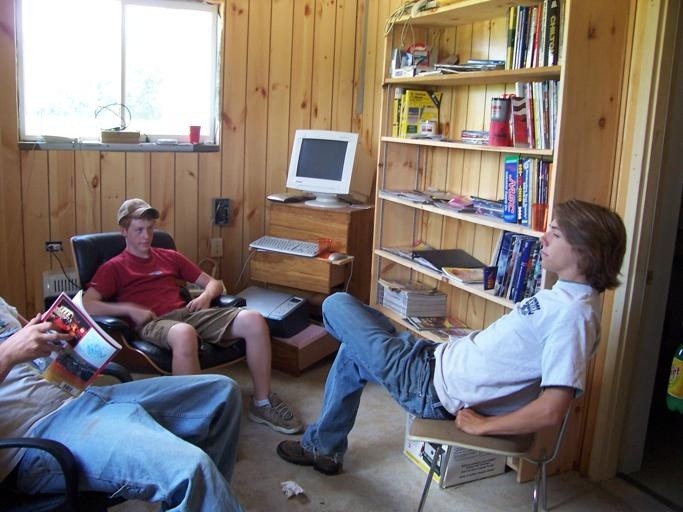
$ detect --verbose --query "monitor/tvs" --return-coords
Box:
[286,130,360,208]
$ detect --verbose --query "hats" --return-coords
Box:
[116,197,160,226]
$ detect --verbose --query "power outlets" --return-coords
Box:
[46,242,62,252]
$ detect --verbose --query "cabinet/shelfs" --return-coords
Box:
[249,199,374,376]
[365,0,627,482]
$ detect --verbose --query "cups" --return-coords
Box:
[316,237,332,259]
[531,202,548,231]
[189,125,200,145]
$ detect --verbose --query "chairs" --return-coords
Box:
[69,228,249,382]
[404,333,603,511]
[0,361,131,512]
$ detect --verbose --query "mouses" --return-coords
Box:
[328,252,347,262]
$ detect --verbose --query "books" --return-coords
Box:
[511,79,561,150]
[504,155,553,231]
[379,187,504,218]
[505,1,565,68]
[485,231,544,303]
[434,59,505,73]
[392,87,442,138]
[379,279,480,341]
[26,290,122,397]
[381,241,484,283]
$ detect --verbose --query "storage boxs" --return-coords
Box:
[398,410,508,488]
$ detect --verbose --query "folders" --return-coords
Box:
[412,248,485,274]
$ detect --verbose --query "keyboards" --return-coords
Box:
[248,235,323,258]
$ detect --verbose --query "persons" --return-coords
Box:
[277,199,626,475]
[0,296,248,512]
[82,197,303,434]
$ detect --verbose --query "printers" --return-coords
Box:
[234,286,311,339]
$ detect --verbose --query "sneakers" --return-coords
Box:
[276,439,344,477]
[246,391,304,436]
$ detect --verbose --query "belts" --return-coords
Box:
[426,340,458,424]
